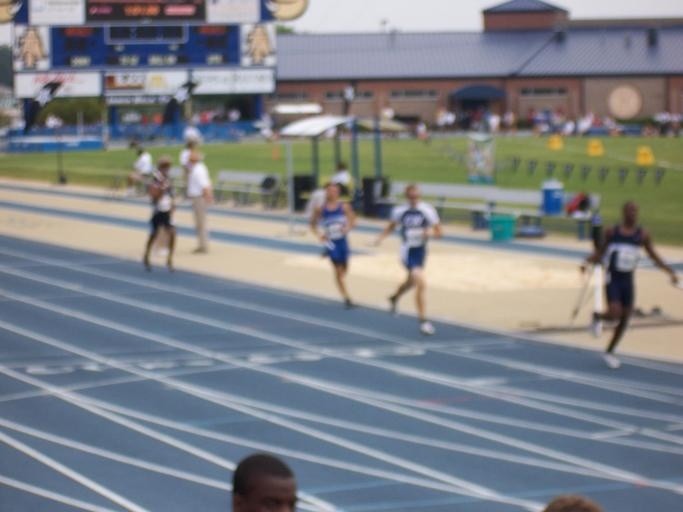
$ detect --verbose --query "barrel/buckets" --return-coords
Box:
[540,181,565,216]
[489,213,516,241]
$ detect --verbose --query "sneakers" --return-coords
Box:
[590,310,603,339]
[600,351,624,370]
[419,319,436,335]
[390,296,398,319]
[342,299,357,310]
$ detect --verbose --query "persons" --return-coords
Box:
[542,496,602,512]
[435,105,615,137]
[310,183,356,310]
[232,454,297,512]
[179,139,197,196]
[42,110,62,130]
[645,110,683,137]
[186,150,214,255]
[116,107,243,125]
[578,200,683,369]
[304,182,332,219]
[372,185,444,334]
[126,148,153,192]
[143,159,179,272]
[327,163,350,195]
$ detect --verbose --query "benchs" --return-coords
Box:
[372,181,603,238]
[209,169,281,209]
